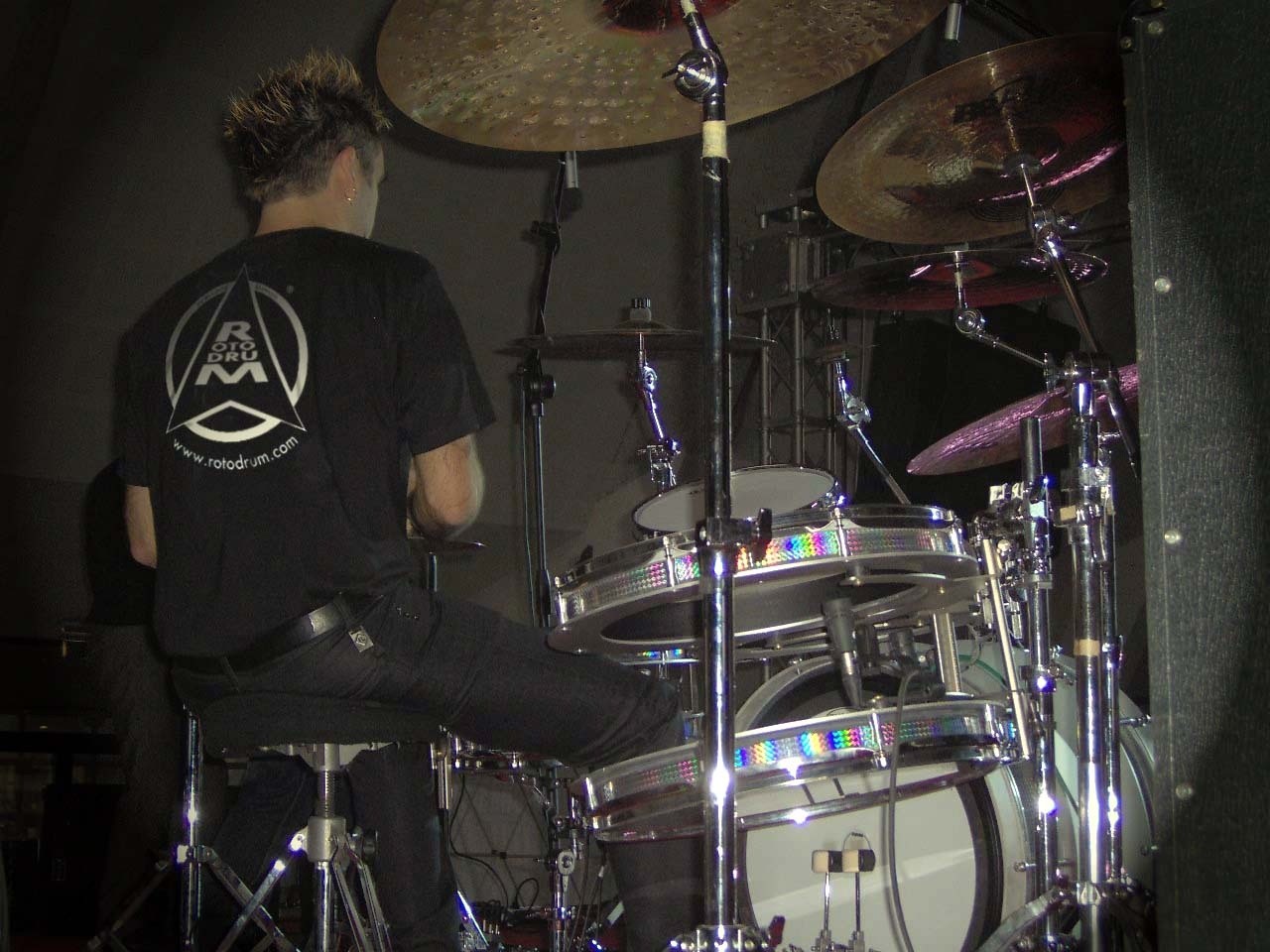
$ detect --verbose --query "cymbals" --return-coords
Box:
[808,249,1112,312]
[816,30,1133,244]
[904,359,1138,476]
[377,0,945,150]
[407,532,483,557]
[500,327,776,364]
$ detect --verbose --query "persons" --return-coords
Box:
[117,48,709,951]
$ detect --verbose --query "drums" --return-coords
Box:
[628,460,842,541]
[721,640,1151,952]
[547,505,984,658]
[570,700,1018,843]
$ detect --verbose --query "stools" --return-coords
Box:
[198,693,439,952]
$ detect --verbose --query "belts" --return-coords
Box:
[163,552,427,684]
